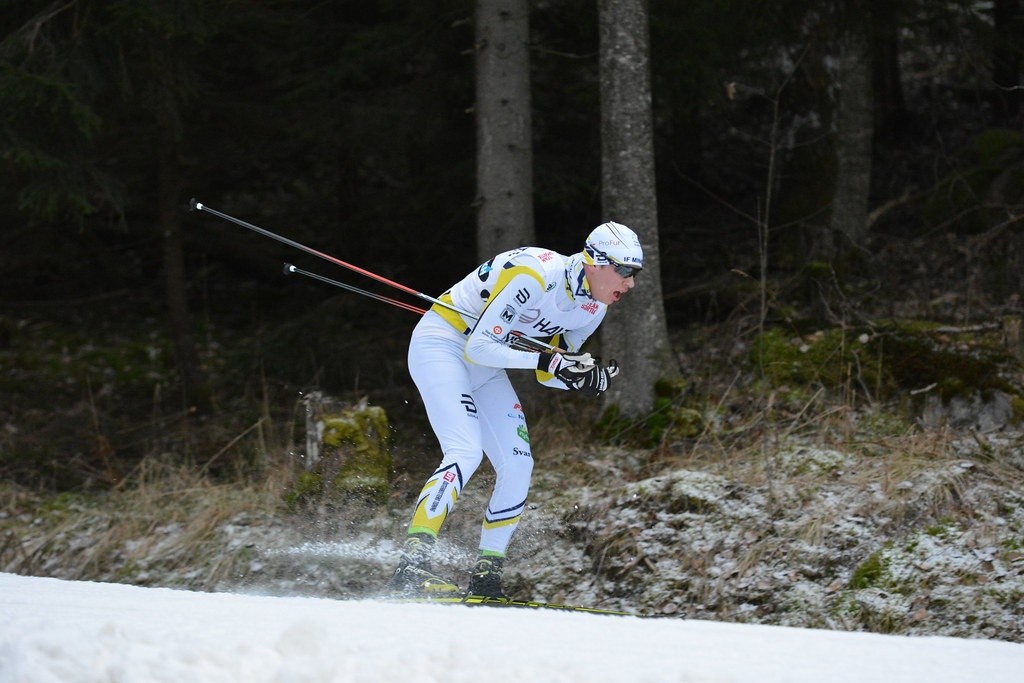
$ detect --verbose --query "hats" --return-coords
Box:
[582,220,643,269]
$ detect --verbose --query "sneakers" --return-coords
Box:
[380,533,460,598]
[463,555,506,599]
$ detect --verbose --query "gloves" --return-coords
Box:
[537,351,602,385]
[565,359,619,400]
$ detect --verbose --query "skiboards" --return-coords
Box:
[343,592,687,621]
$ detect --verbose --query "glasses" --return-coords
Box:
[590,244,643,278]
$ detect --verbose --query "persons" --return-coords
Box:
[389,219,647,608]
